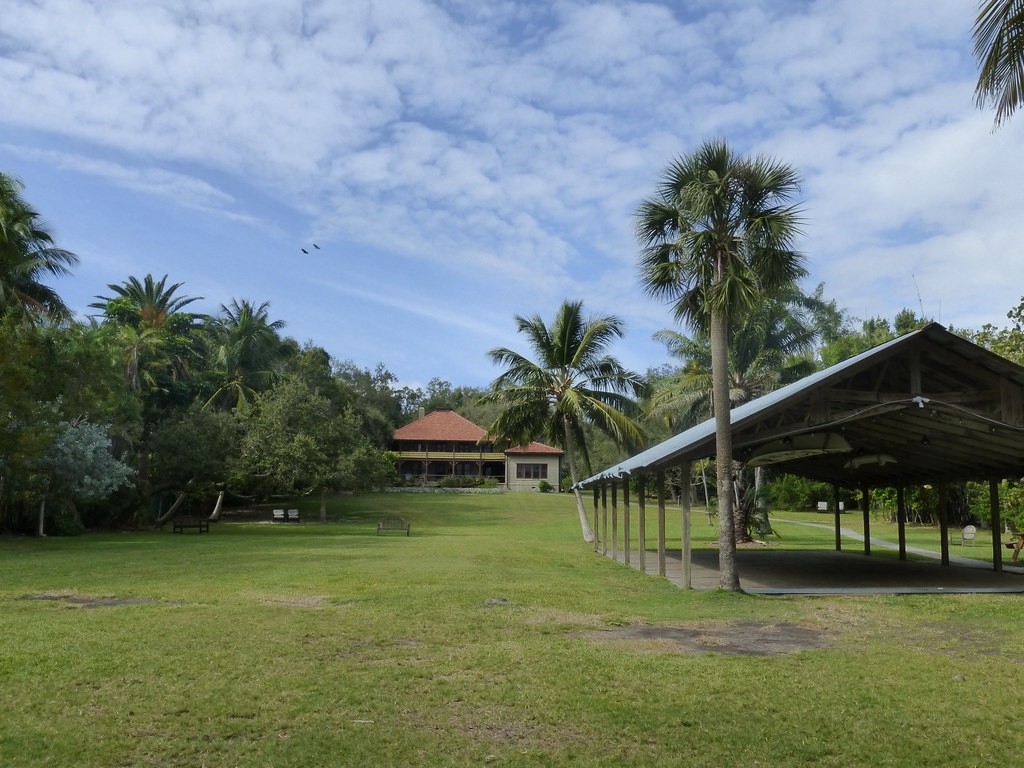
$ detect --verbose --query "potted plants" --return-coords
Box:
[538,479,551,493]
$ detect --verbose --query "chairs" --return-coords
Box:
[839,501,845,514]
[405,473,412,481]
[272,509,286,523]
[816,501,830,513]
[418,472,425,482]
[950,525,976,547]
[287,509,299,523]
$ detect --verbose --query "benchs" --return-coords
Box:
[1005,533,1024,562]
[173,519,209,534]
[376,517,411,536]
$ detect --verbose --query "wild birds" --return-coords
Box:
[300,243,321,255]
